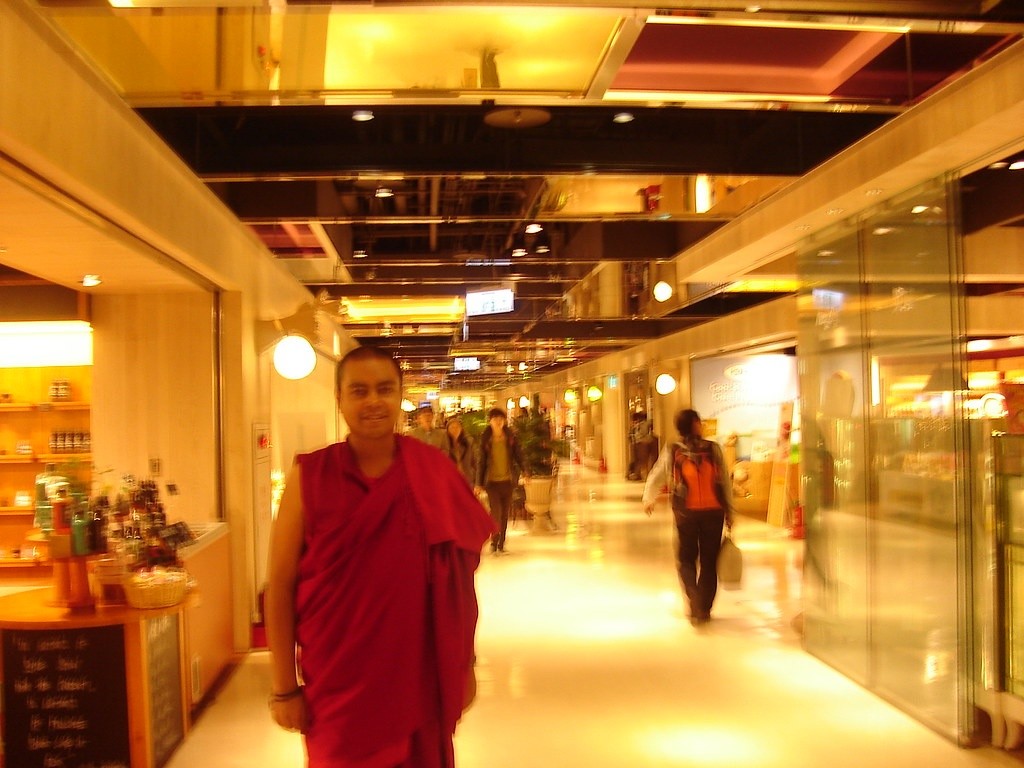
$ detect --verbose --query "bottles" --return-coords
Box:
[32,461,106,554]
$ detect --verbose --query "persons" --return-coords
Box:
[265,345,503,768]
[446,418,475,491]
[641,408,734,625]
[477,408,529,553]
[626,412,652,479]
[406,407,450,457]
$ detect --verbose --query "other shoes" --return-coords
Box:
[688,609,712,626]
[491,541,508,556]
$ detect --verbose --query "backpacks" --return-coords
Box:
[671,446,726,518]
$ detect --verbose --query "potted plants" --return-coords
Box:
[510,404,577,515]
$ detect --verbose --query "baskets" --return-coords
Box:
[124,567,188,610]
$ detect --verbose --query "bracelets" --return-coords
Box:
[270,685,303,702]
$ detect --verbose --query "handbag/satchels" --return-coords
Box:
[717,536,744,590]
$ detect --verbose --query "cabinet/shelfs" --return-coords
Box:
[0,401,92,570]
